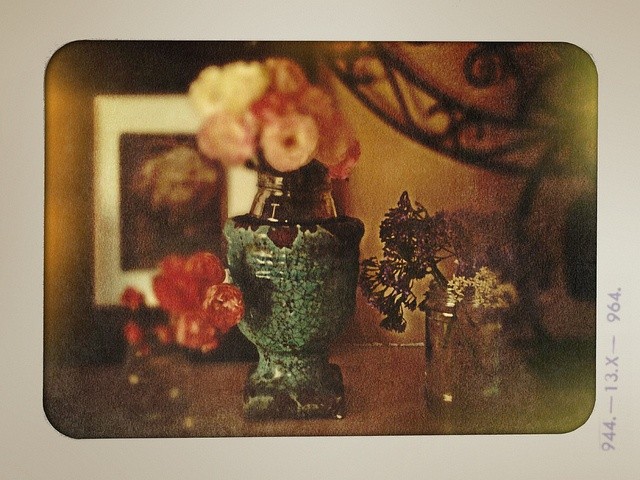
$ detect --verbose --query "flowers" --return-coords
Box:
[116,252,243,354]
[359,196,545,348]
[190,59,359,180]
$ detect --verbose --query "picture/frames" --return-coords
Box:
[94,96,258,311]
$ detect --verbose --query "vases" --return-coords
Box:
[417,287,542,416]
[206,175,364,416]
[121,344,186,423]
